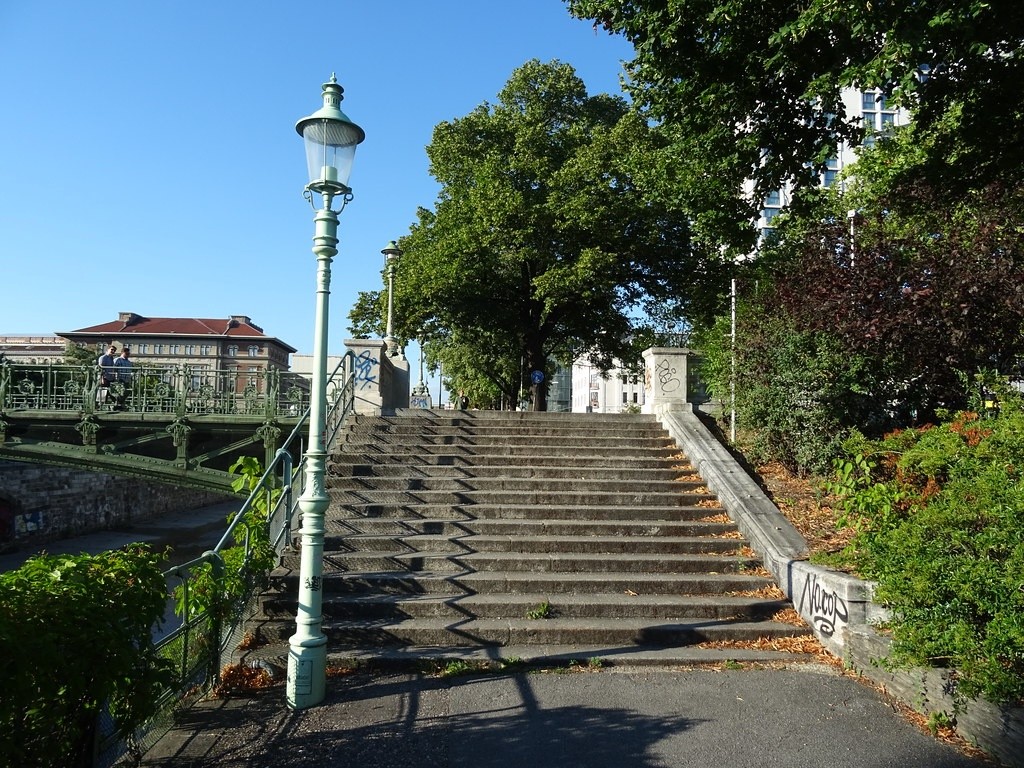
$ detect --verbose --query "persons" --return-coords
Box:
[452,390,469,411]
[95,345,117,409]
[113,347,137,411]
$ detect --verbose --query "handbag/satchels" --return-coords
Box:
[96,386,108,403]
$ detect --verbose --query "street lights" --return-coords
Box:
[415,326,429,397]
[722,263,741,441]
[288,71,368,708]
[381,241,405,354]
